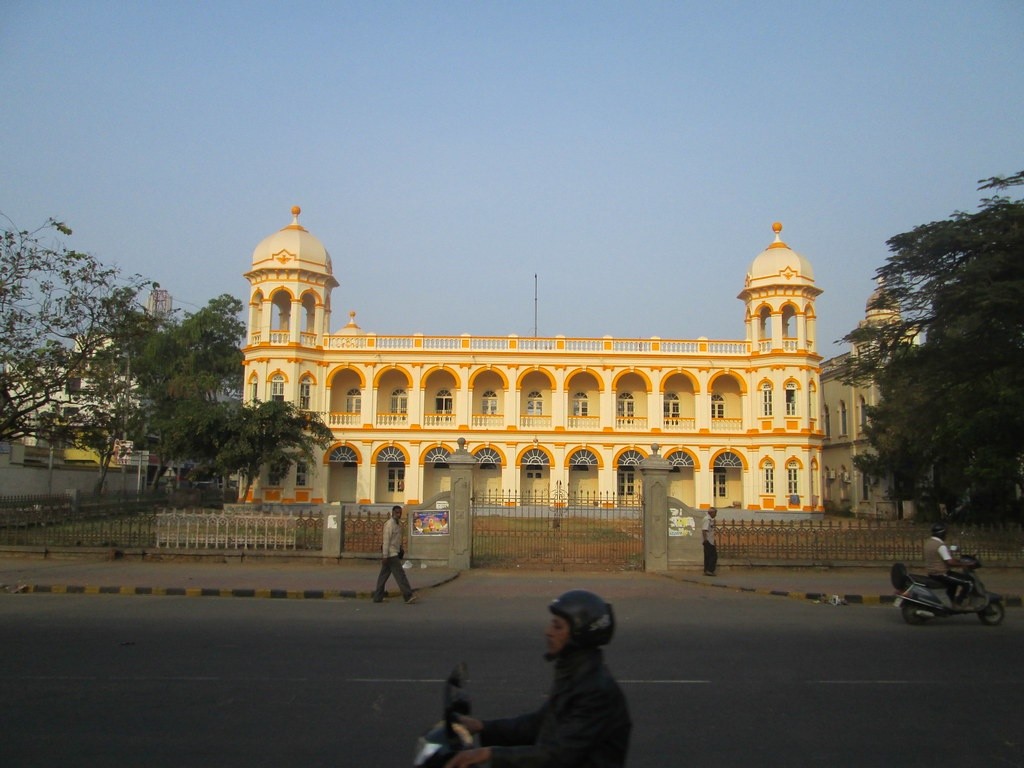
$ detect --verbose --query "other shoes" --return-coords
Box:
[954,602,975,610]
[405,596,418,604]
[706,571,716,576]
[373,598,382,602]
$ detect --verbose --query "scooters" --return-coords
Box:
[891,545,1007,626]
[409,659,485,768]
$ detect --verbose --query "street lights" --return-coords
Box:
[118,350,132,512]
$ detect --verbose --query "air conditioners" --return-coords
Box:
[826,471,835,479]
[841,471,849,483]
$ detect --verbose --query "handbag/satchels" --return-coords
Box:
[398,547,404,560]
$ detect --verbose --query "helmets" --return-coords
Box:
[548,589,614,648]
[932,522,946,537]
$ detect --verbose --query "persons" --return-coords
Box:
[374,505,417,604]
[923,522,975,607]
[701,507,718,576]
[445,590,633,768]
